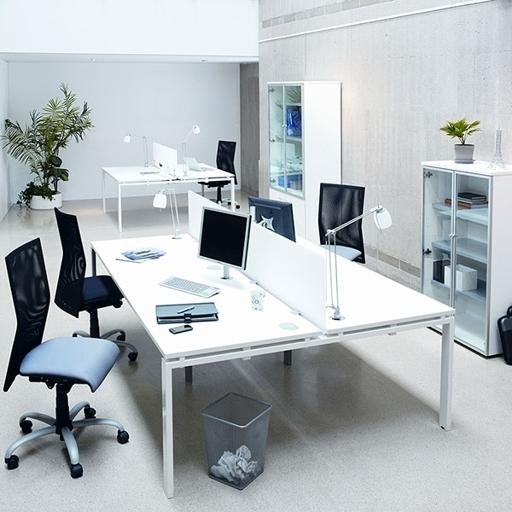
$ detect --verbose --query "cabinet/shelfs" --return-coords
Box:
[420,158,512,358]
[266,81,342,245]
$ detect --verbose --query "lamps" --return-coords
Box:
[320,199,397,326]
[124,125,200,174]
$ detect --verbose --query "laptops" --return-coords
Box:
[184,158,212,172]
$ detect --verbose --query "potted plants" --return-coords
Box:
[440,117,480,164]
[0,82,95,210]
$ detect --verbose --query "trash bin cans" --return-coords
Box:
[199,391,272,490]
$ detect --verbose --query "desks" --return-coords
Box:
[100,165,238,233]
[91,220,455,499]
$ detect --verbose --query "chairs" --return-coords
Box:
[3,207,138,478]
[198,140,240,209]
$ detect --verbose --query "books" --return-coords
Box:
[278,174,301,190]
[433,260,478,291]
[115,246,166,263]
[445,191,488,209]
[286,107,301,137]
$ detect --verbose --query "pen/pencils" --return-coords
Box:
[177,306,195,314]
[136,249,150,255]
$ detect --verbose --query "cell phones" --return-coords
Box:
[169,325,192,334]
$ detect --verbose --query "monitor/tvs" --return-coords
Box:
[197,206,252,271]
[248,196,296,242]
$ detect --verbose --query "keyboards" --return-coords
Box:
[160,276,220,298]
